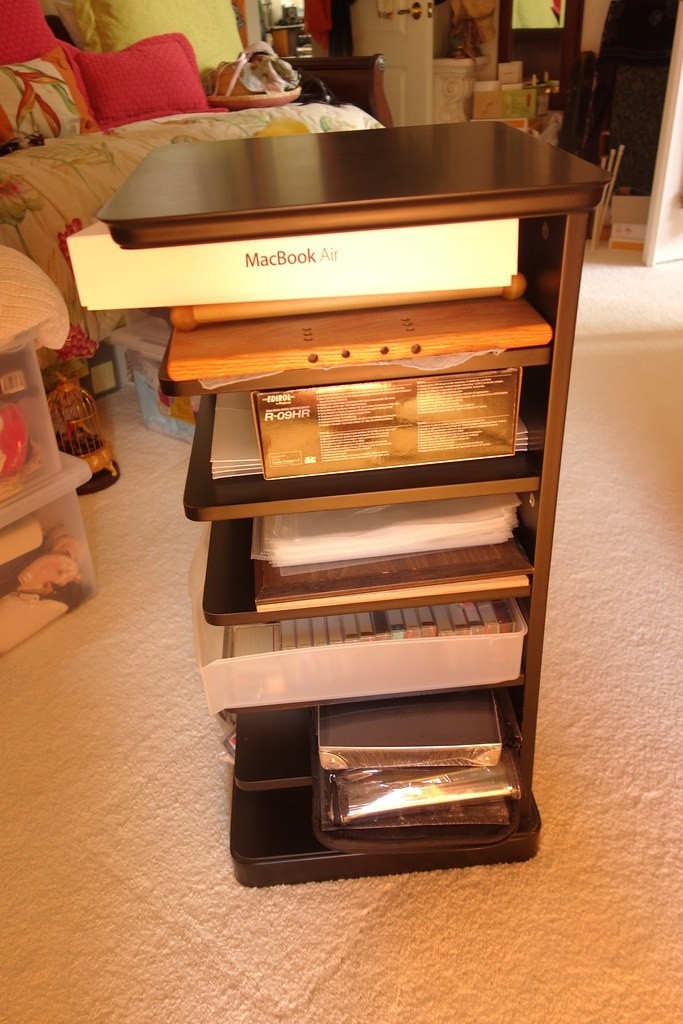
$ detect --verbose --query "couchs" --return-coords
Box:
[0,14,394,371]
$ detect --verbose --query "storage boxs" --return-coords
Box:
[250,367,523,480]
[187,522,529,716]
[473,91,502,119]
[609,187,650,251]
[42,340,129,401]
[65,219,521,311]
[504,117,544,136]
[110,315,200,443]
[0,451,98,657]
[503,86,549,118]
[0,326,63,508]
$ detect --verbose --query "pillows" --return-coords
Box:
[0,0,59,64]
[59,31,229,130]
[0,46,100,154]
[72,0,245,79]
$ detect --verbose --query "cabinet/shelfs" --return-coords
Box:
[96,124,614,887]
